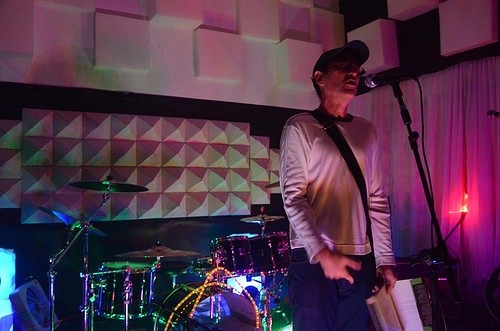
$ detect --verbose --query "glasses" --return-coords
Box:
[321,63,362,75]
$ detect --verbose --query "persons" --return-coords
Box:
[280,40,397,331]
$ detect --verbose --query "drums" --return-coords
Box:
[191,255,214,280]
[209,236,254,272]
[248,231,291,274]
[154,281,261,330]
[93,262,155,320]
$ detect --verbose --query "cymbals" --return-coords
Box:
[240,215,283,223]
[114,247,200,257]
[37,204,110,240]
[68,180,149,193]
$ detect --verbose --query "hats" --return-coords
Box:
[312,40,370,79]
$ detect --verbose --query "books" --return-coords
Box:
[365,277,433,331]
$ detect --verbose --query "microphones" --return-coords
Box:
[364,73,415,88]
